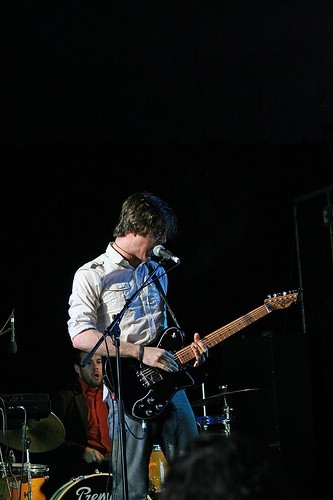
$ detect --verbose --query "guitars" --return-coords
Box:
[102,289,300,420]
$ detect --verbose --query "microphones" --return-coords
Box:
[153,244,180,263]
[8,313,18,353]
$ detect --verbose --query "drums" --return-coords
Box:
[52,472,152,500]
[0,461,50,500]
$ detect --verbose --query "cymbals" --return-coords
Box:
[0,411,66,454]
[190,383,258,407]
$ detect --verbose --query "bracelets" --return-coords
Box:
[139,345,144,364]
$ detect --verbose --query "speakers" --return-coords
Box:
[292,185,333,336]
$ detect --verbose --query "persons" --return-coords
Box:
[51,351,120,474]
[67,192,207,500]
[153,431,262,500]
